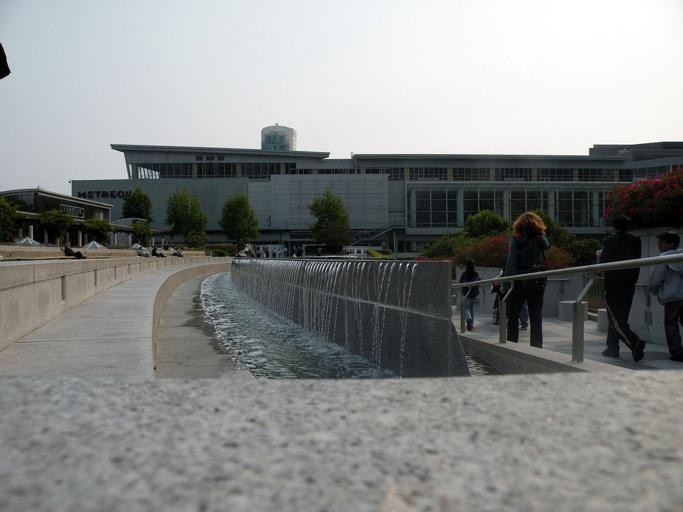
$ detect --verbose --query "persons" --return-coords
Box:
[231,243,365,259]
[498,253,528,331]
[501,210,551,349]
[173,248,184,258]
[647,231,682,364]
[455,257,481,332]
[151,245,168,257]
[489,269,504,326]
[595,213,645,363]
[136,246,149,257]
[63,241,88,260]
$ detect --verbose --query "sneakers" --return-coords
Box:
[602,339,646,362]
[467,319,474,331]
